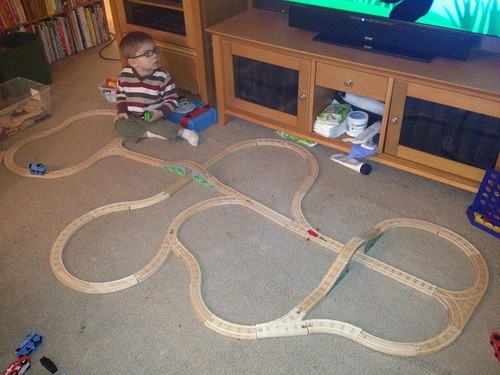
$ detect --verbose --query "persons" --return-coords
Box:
[115,31,199,148]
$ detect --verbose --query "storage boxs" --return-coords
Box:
[0,78,51,143]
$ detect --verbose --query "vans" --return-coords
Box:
[29,163,46,175]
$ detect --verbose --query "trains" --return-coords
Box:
[15,332,43,359]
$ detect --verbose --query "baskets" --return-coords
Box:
[467,169,500,240]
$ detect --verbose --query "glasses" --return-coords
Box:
[132,47,161,59]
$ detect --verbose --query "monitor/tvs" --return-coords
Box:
[279,0,500,64]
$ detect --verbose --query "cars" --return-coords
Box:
[490,331,500,359]
[141,110,153,121]
[2,355,31,375]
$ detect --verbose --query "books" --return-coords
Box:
[0,0,109,64]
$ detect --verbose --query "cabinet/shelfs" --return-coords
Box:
[0,0,112,66]
[204,8,500,195]
[105,0,255,107]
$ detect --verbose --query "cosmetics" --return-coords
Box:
[330,153,372,175]
